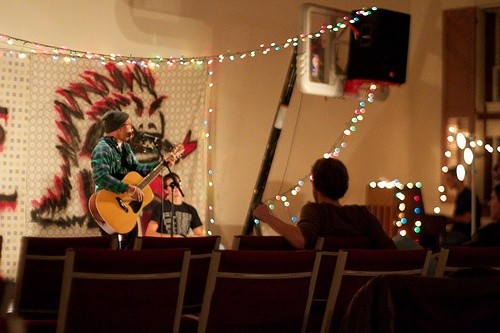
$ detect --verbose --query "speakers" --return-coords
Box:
[346,8,410,85]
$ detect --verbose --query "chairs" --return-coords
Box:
[0,235,500,333]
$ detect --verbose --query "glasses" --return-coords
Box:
[119,122,133,129]
[308,175,314,183]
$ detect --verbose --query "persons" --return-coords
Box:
[251,158,399,299]
[90,109,178,253]
[144,173,205,238]
[432,166,500,254]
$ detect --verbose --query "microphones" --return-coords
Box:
[141,133,160,142]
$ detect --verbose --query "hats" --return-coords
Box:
[101,110,129,133]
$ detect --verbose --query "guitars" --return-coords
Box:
[88,142,186,236]
